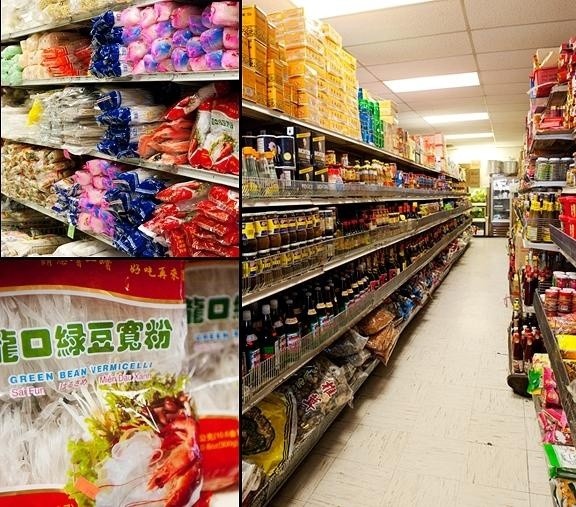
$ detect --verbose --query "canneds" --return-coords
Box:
[388,211,400,224]
[326,149,379,184]
[544,271,576,318]
[242,207,337,296]
[535,157,576,187]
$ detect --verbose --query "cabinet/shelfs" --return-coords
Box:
[0,0,576,507]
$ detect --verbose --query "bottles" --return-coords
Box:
[239,200,419,392]
[510,191,563,374]
